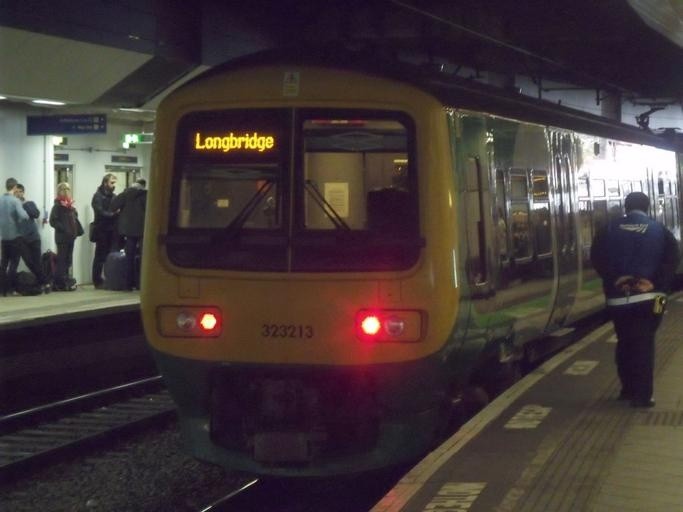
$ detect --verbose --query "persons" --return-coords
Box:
[0,177,29,296]
[91,174,120,289]
[111,179,147,291]
[15,184,51,290]
[590,192,680,407]
[49,182,78,291]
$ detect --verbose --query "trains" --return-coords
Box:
[139,48,681,483]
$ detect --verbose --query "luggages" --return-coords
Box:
[106,251,128,290]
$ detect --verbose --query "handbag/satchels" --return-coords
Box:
[43,252,57,288]
[89,223,113,245]
[16,271,42,295]
